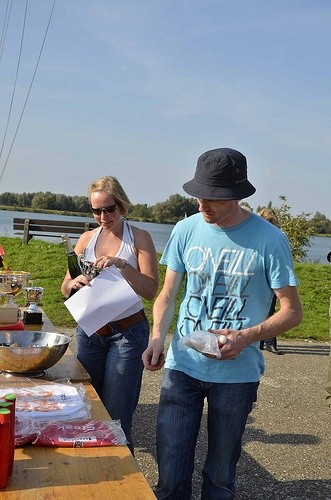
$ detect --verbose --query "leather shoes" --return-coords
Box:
[266,345,283,355]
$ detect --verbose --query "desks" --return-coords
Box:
[0,305,92,382]
[0,382,159,500]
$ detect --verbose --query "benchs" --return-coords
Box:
[13,218,100,245]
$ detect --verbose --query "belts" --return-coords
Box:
[93,307,146,337]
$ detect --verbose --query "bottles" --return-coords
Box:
[61,234,83,279]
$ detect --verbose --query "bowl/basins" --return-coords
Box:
[0,331,72,378]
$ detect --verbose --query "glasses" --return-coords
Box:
[91,204,118,215]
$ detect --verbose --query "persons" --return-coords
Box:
[61,176,160,460]
[141,148,302,500]
[257,208,285,355]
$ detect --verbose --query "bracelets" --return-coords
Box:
[122,259,129,268]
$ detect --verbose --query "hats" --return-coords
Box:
[326,251,331,262]
[182,148,256,200]
